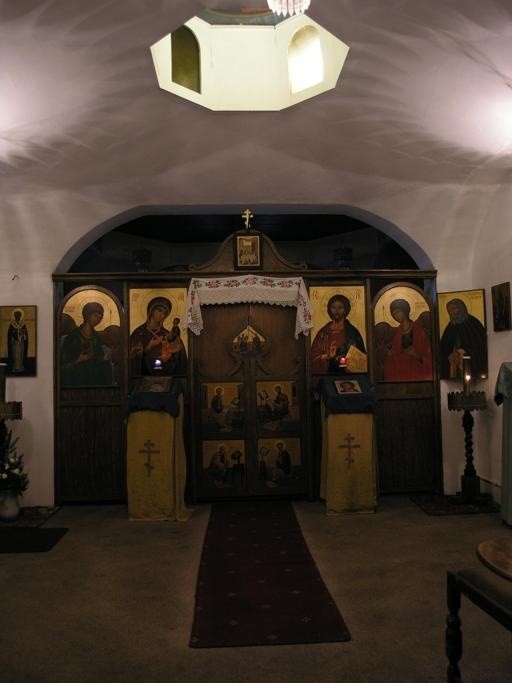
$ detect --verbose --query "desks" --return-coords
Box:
[444,560,512,683]
[493,361,510,528]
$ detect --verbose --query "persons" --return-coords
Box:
[273,442,291,476]
[271,385,289,416]
[130,296,189,379]
[211,386,225,417]
[339,381,358,393]
[378,298,433,381]
[60,302,115,385]
[208,443,226,488]
[438,298,487,378]
[240,247,248,255]
[307,295,366,375]
[7,311,28,372]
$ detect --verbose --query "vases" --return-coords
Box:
[1,489,19,521]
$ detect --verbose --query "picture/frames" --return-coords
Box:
[56,284,124,389]
[307,281,369,375]
[129,288,189,394]
[436,289,490,380]
[1,305,37,378]
[370,281,436,384]
[200,381,302,495]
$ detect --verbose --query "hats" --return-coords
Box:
[149,297,172,318]
[391,299,410,313]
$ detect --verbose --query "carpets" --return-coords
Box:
[1,507,60,528]
[0,527,69,553]
[189,500,351,647]
